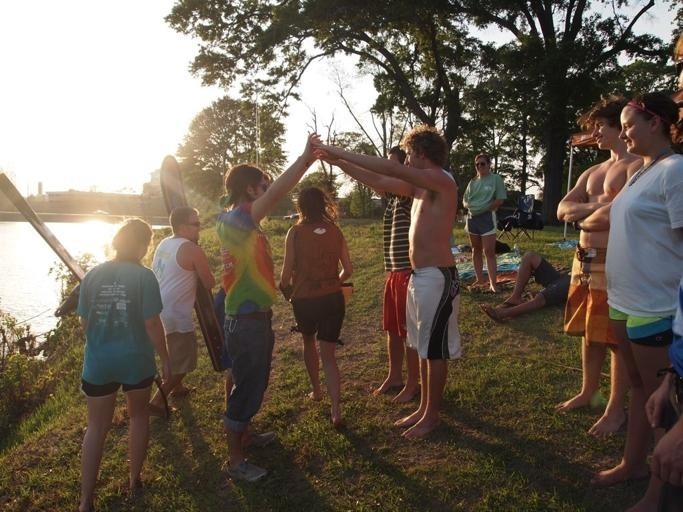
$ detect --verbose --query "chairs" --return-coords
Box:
[496,193,546,241]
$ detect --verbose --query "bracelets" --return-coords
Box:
[297,157,309,171]
[654,367,679,381]
[571,220,578,232]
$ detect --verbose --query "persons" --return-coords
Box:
[587,94,683,510]
[372,144,419,405]
[478,249,571,321]
[299,158,508,255]
[216,132,323,484]
[553,94,644,442]
[460,155,508,294]
[280,186,354,428]
[306,122,462,443]
[78,216,173,511]
[149,205,215,417]
[647,31,683,512]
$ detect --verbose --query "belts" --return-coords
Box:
[234,309,273,320]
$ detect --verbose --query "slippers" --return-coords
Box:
[479,304,506,324]
[495,302,523,308]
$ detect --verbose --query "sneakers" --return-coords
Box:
[221,460,267,483]
[146,402,177,417]
[170,386,196,398]
[244,432,277,449]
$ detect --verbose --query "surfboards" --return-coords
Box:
[160,155,232,372]
[0,172,86,282]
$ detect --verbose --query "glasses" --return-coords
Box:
[476,162,486,167]
